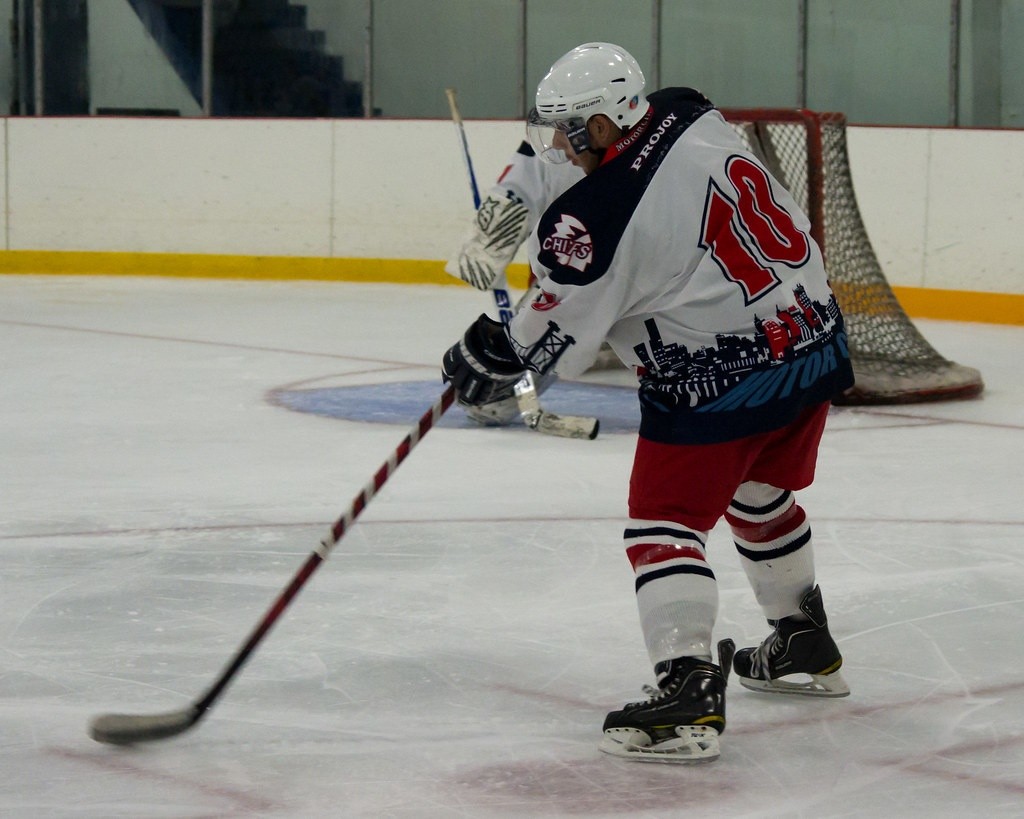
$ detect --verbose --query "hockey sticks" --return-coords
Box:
[444,86,602,439]
[90,385,457,743]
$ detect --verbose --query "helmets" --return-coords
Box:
[535,41,650,129]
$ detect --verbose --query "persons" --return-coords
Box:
[439,41,856,766]
[443,106,587,294]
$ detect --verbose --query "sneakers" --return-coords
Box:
[733,588,850,697]
[599,636,734,764]
[465,398,522,425]
